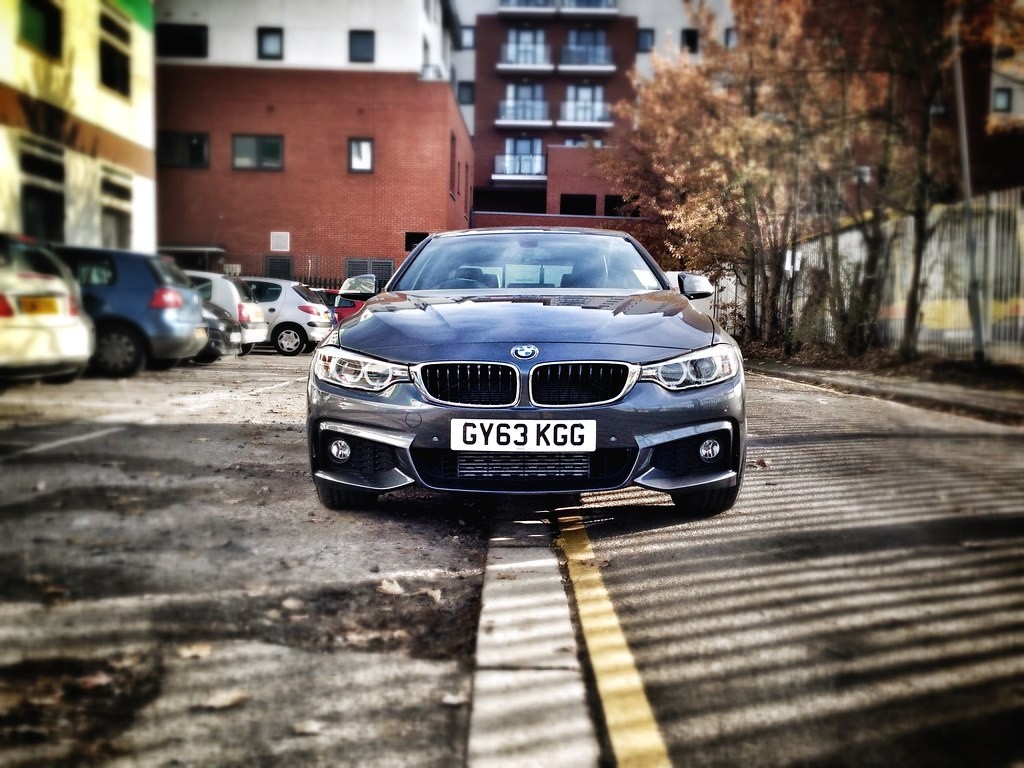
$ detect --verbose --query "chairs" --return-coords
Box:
[453,267,499,287]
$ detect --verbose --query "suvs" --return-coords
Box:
[49,244,208,379]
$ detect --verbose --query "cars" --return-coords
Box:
[305,226,748,517]
[0,231,95,394]
[181,269,375,364]
